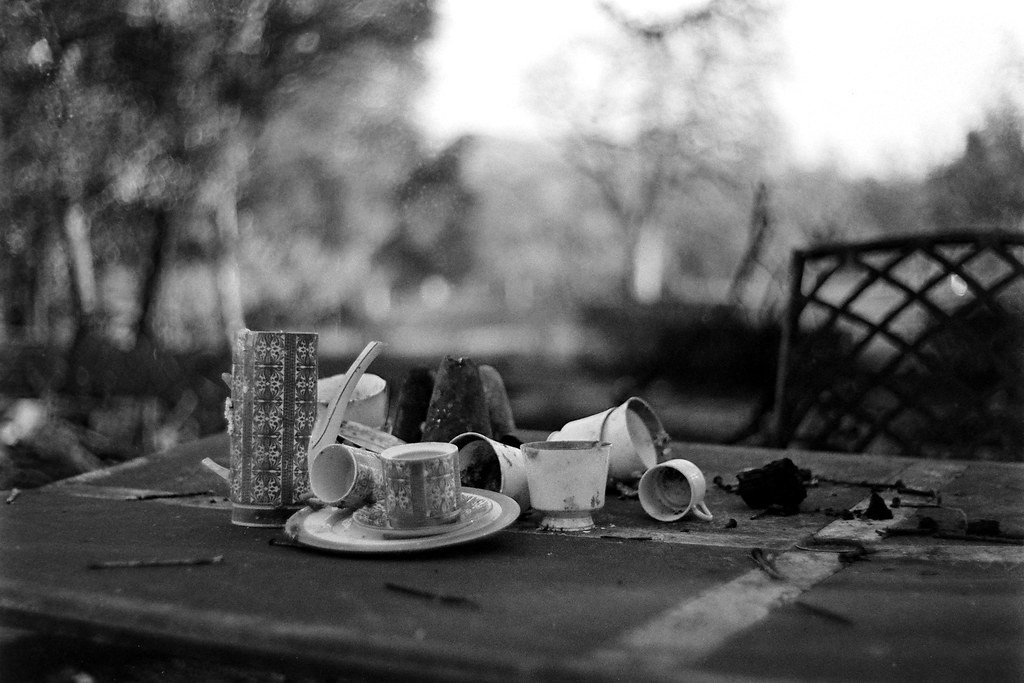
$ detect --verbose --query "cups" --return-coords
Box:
[635,459,716,525]
[447,433,533,515]
[517,439,613,536]
[546,409,609,445]
[600,395,673,481]
[313,371,389,433]
[225,330,319,532]
[378,438,466,530]
[310,444,389,511]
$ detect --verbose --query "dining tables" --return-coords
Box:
[0,424,1023,683]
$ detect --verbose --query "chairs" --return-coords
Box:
[742,226,1023,462]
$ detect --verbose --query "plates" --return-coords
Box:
[354,493,496,539]
[284,486,524,557]
